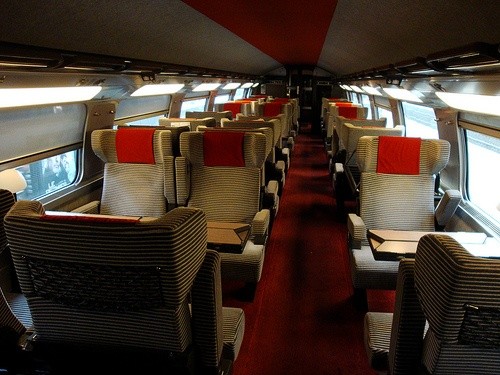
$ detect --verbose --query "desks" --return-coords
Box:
[367,228,500,259]
[124,216,252,254]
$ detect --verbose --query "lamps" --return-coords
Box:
[0,71,260,109]
[340,85,500,116]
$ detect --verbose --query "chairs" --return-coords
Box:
[321,97,500,375]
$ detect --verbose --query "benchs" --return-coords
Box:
[0,96,300,375]
